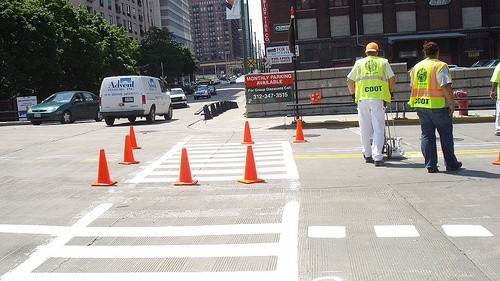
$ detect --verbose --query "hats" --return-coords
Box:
[366,42,379,53]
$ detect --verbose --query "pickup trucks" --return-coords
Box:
[167,87,188,108]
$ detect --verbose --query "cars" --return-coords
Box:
[448,64,458,69]
[27,91,103,125]
[209,85,217,95]
[229,72,242,83]
[185,74,227,90]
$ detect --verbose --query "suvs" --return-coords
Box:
[472,59,500,67]
[194,84,212,99]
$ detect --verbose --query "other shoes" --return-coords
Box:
[427,165,440,173]
[374,159,384,166]
[364,155,373,163]
[445,162,462,171]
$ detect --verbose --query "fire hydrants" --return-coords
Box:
[455,90,470,116]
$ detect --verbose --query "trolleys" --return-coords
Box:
[358,95,394,160]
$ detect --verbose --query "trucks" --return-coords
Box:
[99,75,172,125]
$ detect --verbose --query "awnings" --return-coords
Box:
[388,33,467,43]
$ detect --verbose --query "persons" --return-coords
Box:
[346,42,396,166]
[490,63,500,135]
[407,41,462,173]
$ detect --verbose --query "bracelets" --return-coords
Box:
[492,90,494,91]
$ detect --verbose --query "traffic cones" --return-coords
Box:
[129,125,142,149]
[237,146,265,183]
[293,119,306,143]
[117,135,140,164]
[174,148,198,186]
[241,121,254,145]
[91,149,118,186]
[492,150,500,166]
